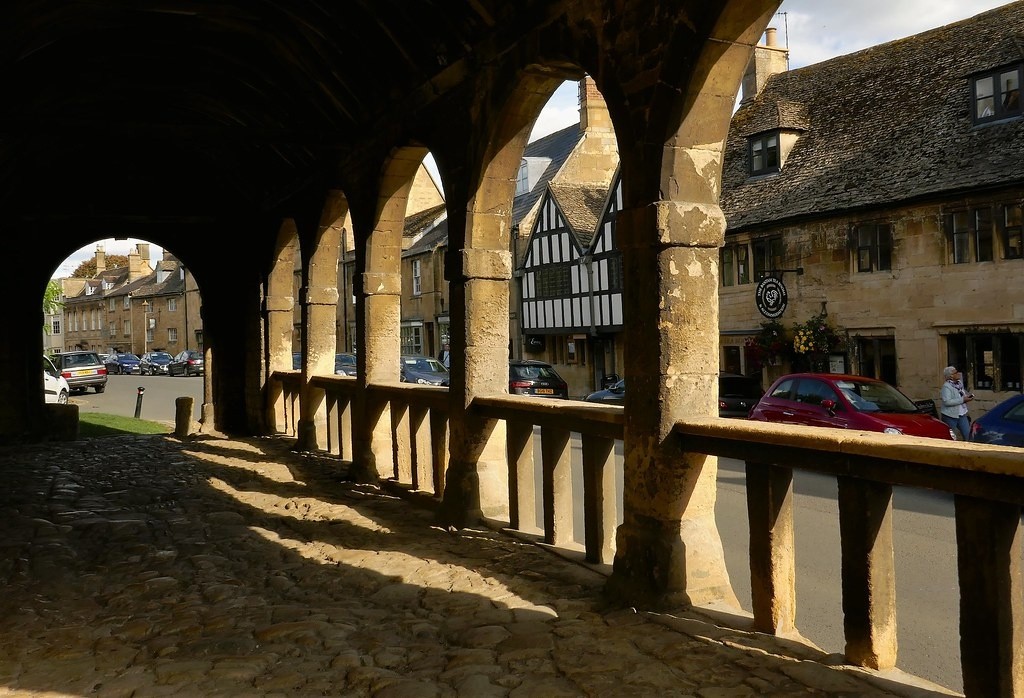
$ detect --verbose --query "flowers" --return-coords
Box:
[791,316,862,360]
[743,319,791,368]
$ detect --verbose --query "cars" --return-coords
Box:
[440,359,569,399]
[49,350,108,395]
[748,373,959,441]
[97,353,110,364]
[168,350,205,377]
[44,353,71,405]
[138,352,174,375]
[104,354,142,376]
[335,352,358,375]
[582,371,764,421]
[400,354,451,386]
[970,395,1024,449]
[292,351,302,370]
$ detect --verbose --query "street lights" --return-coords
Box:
[142,300,151,356]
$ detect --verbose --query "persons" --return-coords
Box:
[438,344,450,369]
[941,366,974,441]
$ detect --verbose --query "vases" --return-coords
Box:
[769,355,781,367]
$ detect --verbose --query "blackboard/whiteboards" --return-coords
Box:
[914,399,939,419]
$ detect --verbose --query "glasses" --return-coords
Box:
[951,371,958,375]
